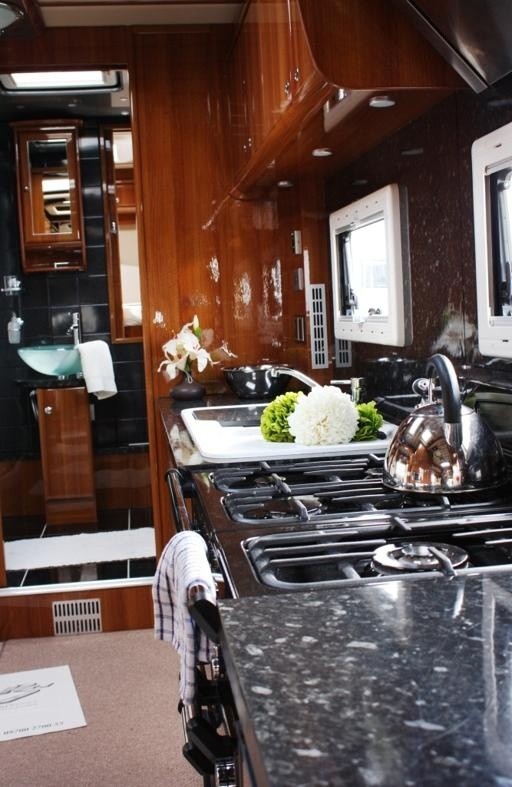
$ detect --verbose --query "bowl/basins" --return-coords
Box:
[220,363,292,397]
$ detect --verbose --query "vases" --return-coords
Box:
[169,372,206,400]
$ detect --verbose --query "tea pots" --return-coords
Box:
[381,354,503,501]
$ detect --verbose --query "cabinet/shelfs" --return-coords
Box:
[36,386,100,525]
[221,1,466,207]
[11,118,88,272]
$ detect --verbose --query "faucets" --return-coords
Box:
[271,367,323,391]
[66,312,83,346]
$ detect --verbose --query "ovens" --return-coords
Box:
[180,635,242,787]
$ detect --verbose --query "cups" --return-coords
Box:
[3,274,17,295]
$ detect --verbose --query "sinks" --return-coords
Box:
[17,345,82,378]
[180,403,389,461]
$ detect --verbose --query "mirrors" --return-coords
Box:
[28,138,73,235]
[98,127,146,343]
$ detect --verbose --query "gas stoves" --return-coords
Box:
[167,446,508,600]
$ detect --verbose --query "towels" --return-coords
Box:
[150,529,217,706]
[75,340,119,400]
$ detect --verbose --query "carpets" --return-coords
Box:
[4,525,154,574]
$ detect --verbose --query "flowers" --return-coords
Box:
[156,313,222,383]
[259,385,387,447]
